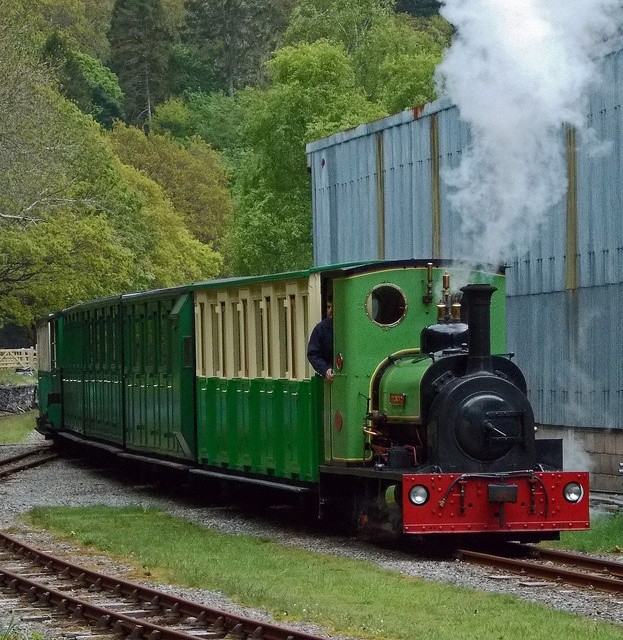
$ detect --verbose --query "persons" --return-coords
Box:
[307,291,335,383]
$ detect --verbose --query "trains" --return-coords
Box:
[28,259,589,556]
